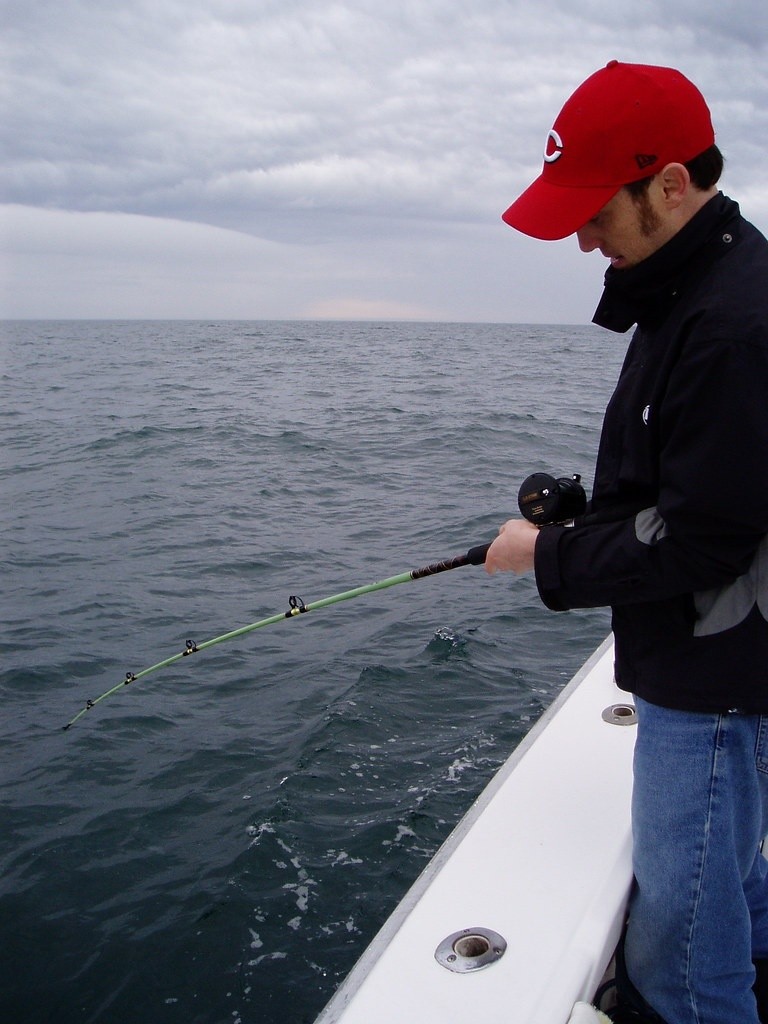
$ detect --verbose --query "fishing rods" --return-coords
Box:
[61,469,588,733]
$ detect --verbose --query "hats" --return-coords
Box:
[502,60,715,240]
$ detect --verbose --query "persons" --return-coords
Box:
[485,60,768,1024]
